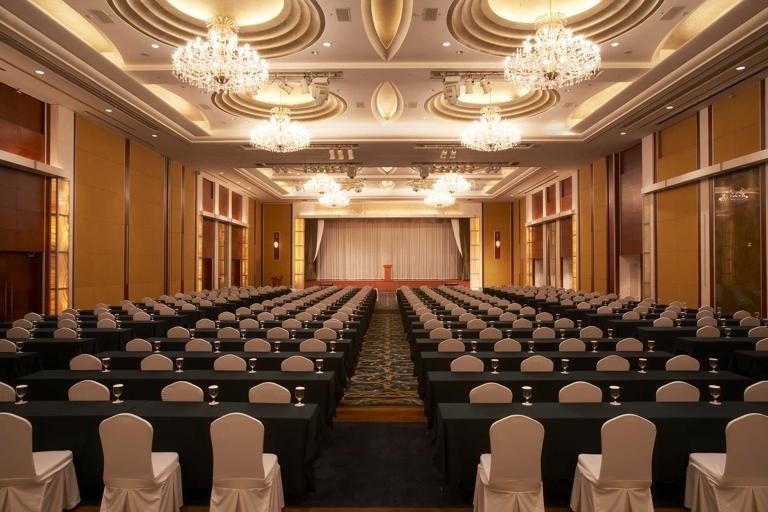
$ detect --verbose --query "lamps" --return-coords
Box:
[271,164,363,209]
[460,92,522,152]
[273,238,279,249]
[412,163,503,207]
[249,92,311,153]
[495,238,501,249]
[328,144,354,160]
[271,72,337,106]
[440,146,458,161]
[171,0,269,94]
[441,73,493,106]
[502,0,602,91]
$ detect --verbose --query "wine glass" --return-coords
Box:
[708,384,723,406]
[294,385,305,407]
[11,315,358,374]
[207,385,221,406]
[111,383,124,404]
[521,385,534,406]
[608,386,623,406]
[435,312,766,374]
[13,384,29,405]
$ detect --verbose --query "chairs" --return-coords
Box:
[741,380,767,402]
[686,413,766,511]
[242,383,294,402]
[95,413,188,509]
[398,284,767,379]
[68,383,111,400]
[2,285,377,380]
[556,381,604,402]
[2,383,31,401]
[652,379,702,402]
[468,413,549,511]
[160,379,203,401]
[467,381,513,403]
[2,411,90,508]
[570,413,658,511]
[206,411,287,512]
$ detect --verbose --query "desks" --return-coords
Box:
[438,401,768,457]
[0,402,336,477]
